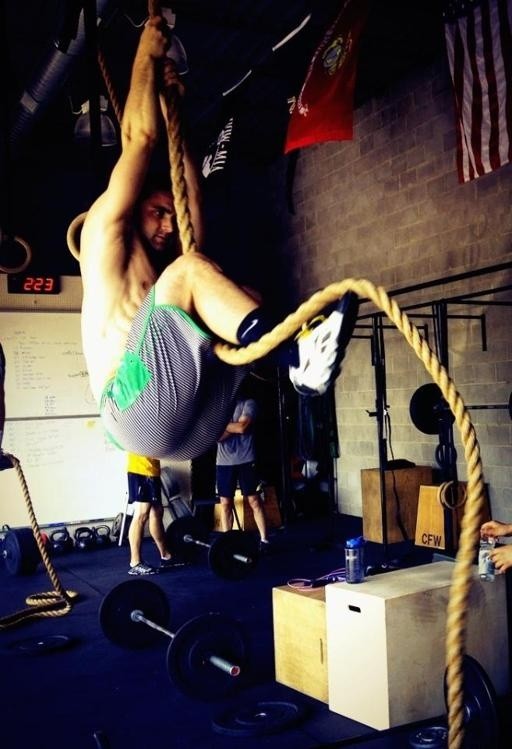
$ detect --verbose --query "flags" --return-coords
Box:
[281,0,368,156]
[199,68,264,181]
[436,0,512,183]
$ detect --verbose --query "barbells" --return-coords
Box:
[100,579,255,700]
[165,517,252,575]
[405,383,512,433]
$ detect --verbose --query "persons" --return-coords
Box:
[214,397,269,544]
[76,12,361,466]
[121,450,172,575]
[478,518,512,573]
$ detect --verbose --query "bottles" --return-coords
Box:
[345,539,363,583]
[478,537,496,583]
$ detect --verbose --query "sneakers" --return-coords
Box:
[127,553,187,576]
[286,288,360,400]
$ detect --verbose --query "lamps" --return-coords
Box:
[74,93,118,149]
